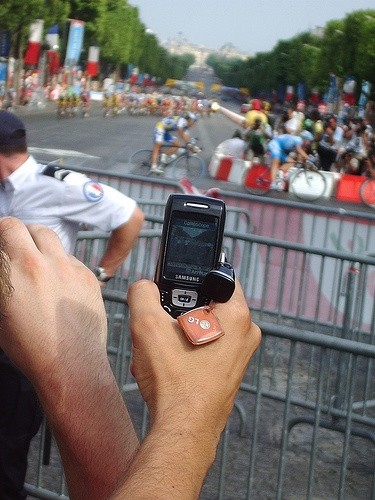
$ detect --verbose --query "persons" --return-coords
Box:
[0,73,375,213]
[1,111,144,499]
[108,272,262,499]
[0,215,143,500]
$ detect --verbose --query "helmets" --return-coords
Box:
[185,111,198,121]
[300,129,315,141]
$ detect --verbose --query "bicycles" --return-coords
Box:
[359,175,375,209]
[128,139,205,183]
[241,149,326,202]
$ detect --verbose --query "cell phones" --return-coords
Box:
[153,192,227,320]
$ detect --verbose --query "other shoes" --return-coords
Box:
[268,183,280,190]
[160,153,168,164]
[150,167,164,175]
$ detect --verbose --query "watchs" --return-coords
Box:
[93,266,113,284]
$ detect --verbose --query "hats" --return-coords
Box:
[0,110,27,144]
